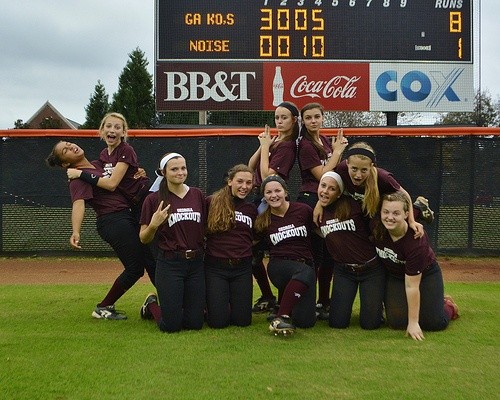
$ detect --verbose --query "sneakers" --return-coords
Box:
[251,295,276,313]
[412,196,435,224]
[269,317,297,337]
[269,303,282,313]
[444,294,462,320]
[316,301,331,320]
[140,292,156,322]
[91,305,127,321]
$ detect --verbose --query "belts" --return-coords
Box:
[208,256,251,264]
[338,261,378,271]
[157,248,205,259]
[283,254,315,267]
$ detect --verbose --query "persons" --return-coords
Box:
[45,141,150,320]
[203,164,259,328]
[137,152,207,333]
[314,141,436,301]
[313,170,387,329]
[247,101,300,314]
[295,102,348,321]
[64,114,159,286]
[250,173,321,337]
[373,190,460,342]
